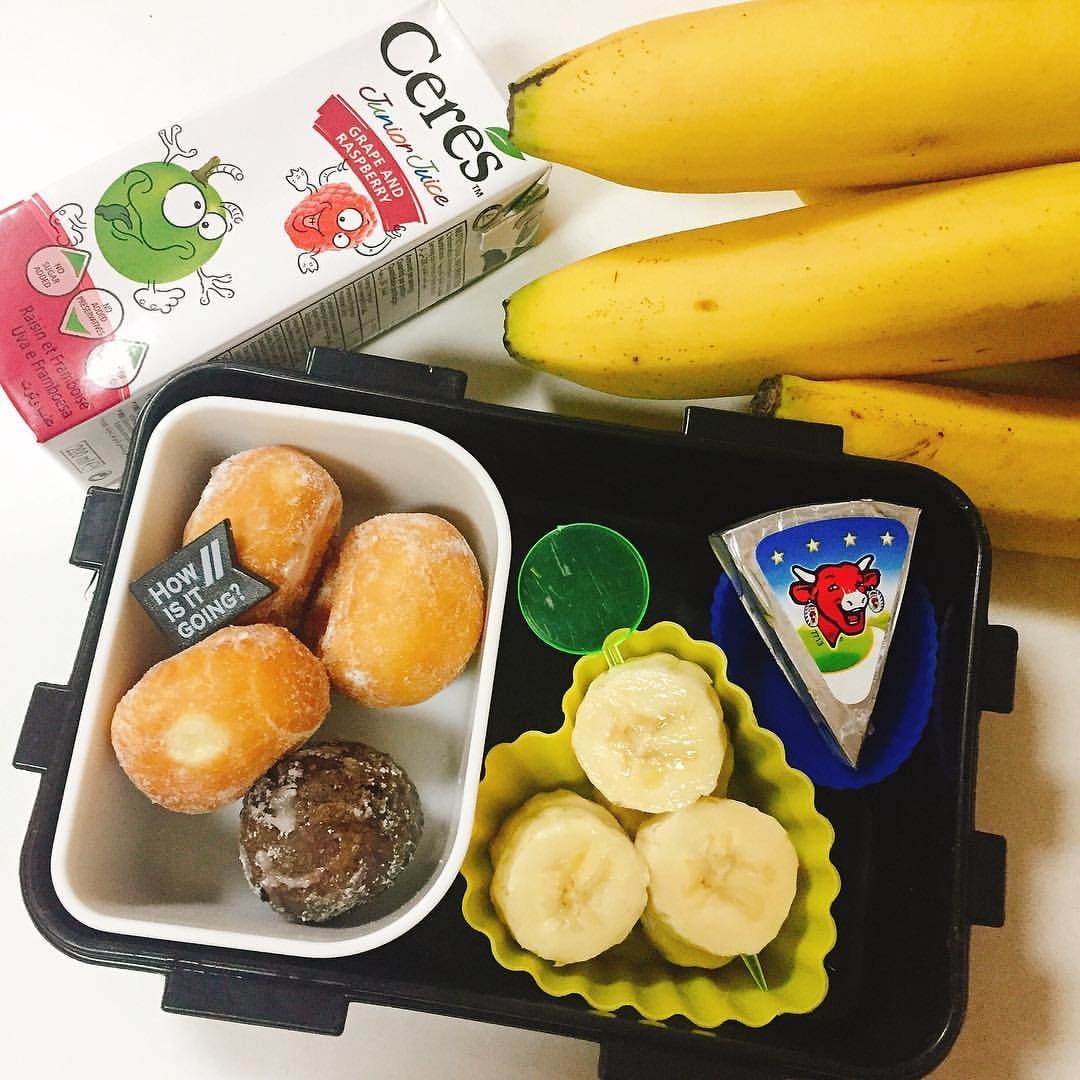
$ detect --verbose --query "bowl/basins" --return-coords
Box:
[457,619,842,1030]
[709,571,938,790]
[50,393,514,961]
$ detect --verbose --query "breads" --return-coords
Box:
[102,444,480,921]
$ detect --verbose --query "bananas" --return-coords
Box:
[488,649,800,971]
[501,0,1079,565]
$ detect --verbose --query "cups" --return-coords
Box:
[517,522,650,655]
